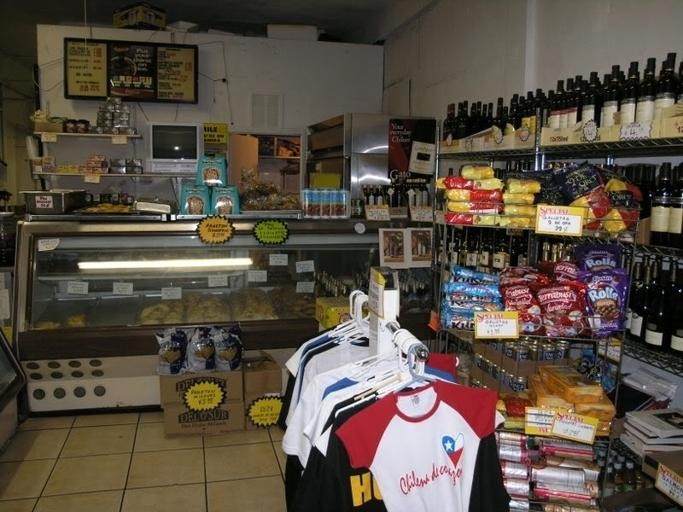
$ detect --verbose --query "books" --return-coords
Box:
[620,408,683,458]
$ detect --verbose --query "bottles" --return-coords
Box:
[360,182,430,207]
[437,51,683,361]
[590,440,656,498]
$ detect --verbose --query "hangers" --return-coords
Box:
[294,290,484,439]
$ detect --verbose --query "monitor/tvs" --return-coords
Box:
[144,121,204,174]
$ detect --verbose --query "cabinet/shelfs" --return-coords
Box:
[10,214,409,417]
[236,132,300,160]
[31,130,196,178]
[431,130,682,510]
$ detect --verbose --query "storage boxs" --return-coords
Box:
[159,355,282,439]
